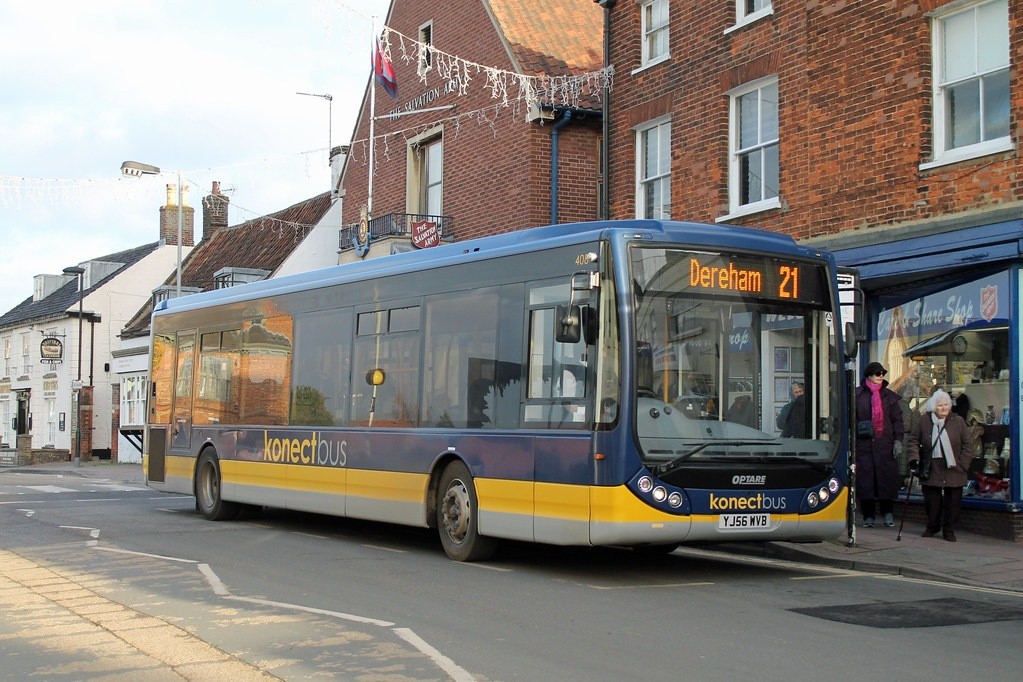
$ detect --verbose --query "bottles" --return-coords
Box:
[1001,406,1010,425]
[999,438,1010,479]
[986,405,994,425]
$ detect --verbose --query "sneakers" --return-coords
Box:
[883,514,894,527]
[863,517,875,528]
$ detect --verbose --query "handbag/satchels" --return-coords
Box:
[858,420,873,436]
[914,452,931,480]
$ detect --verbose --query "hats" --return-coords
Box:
[864,362,887,377]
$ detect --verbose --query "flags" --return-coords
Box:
[371,35,397,99]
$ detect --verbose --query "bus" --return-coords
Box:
[143,218,867,566]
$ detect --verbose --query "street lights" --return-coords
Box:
[62,266,84,467]
[115,159,184,298]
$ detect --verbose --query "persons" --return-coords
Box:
[908,388,973,542]
[855,362,904,526]
[556,341,612,415]
[777,382,805,438]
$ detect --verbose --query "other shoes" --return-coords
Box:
[944,532,956,541]
[922,529,934,537]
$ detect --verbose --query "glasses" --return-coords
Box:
[873,371,885,377]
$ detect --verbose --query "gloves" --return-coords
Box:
[892,440,902,458]
[910,460,919,472]
